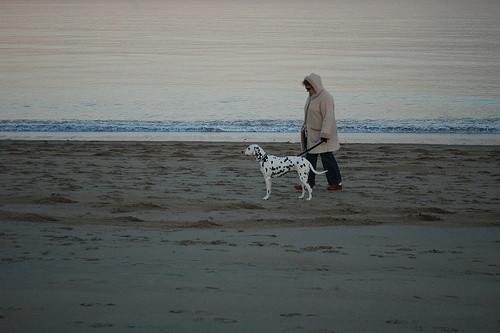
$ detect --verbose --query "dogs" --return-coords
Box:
[242,144,328,201]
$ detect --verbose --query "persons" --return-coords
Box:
[294,72,342,190]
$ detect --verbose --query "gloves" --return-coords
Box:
[321,138,327,142]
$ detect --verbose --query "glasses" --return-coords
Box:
[306,87,312,90]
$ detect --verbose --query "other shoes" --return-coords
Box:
[327,184,342,190]
[295,185,313,189]
